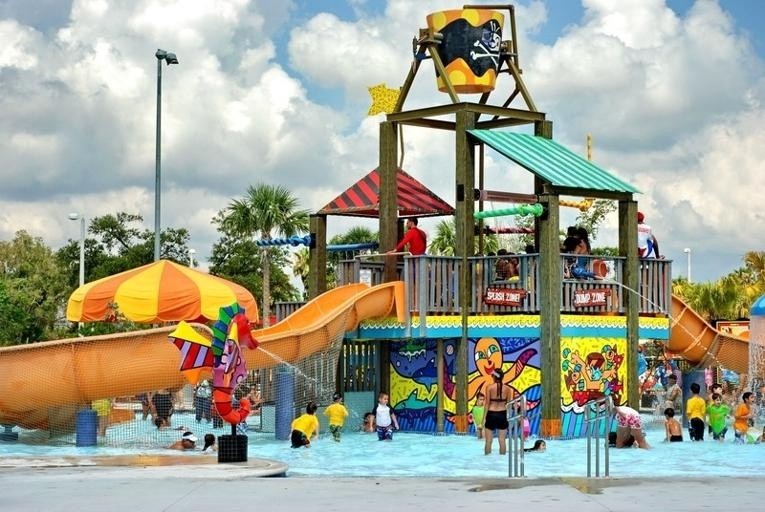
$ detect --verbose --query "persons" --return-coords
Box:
[637,211,667,261]
[432,224,592,315]
[484,368,511,456]
[525,440,547,453]
[138,376,265,436]
[323,392,349,444]
[290,401,319,448]
[385,216,427,280]
[472,393,487,440]
[608,371,765,451]
[521,415,532,443]
[200,434,219,456]
[93,398,116,440]
[371,391,400,441]
[167,431,200,451]
[362,412,377,433]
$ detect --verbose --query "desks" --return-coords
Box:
[187,248,198,270]
[683,245,692,283]
[152,46,179,262]
[68,213,86,287]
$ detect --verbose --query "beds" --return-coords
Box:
[63,256,262,327]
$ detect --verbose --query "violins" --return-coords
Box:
[0,279,407,435]
[663,296,765,375]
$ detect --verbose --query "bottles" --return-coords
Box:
[182,434,199,442]
